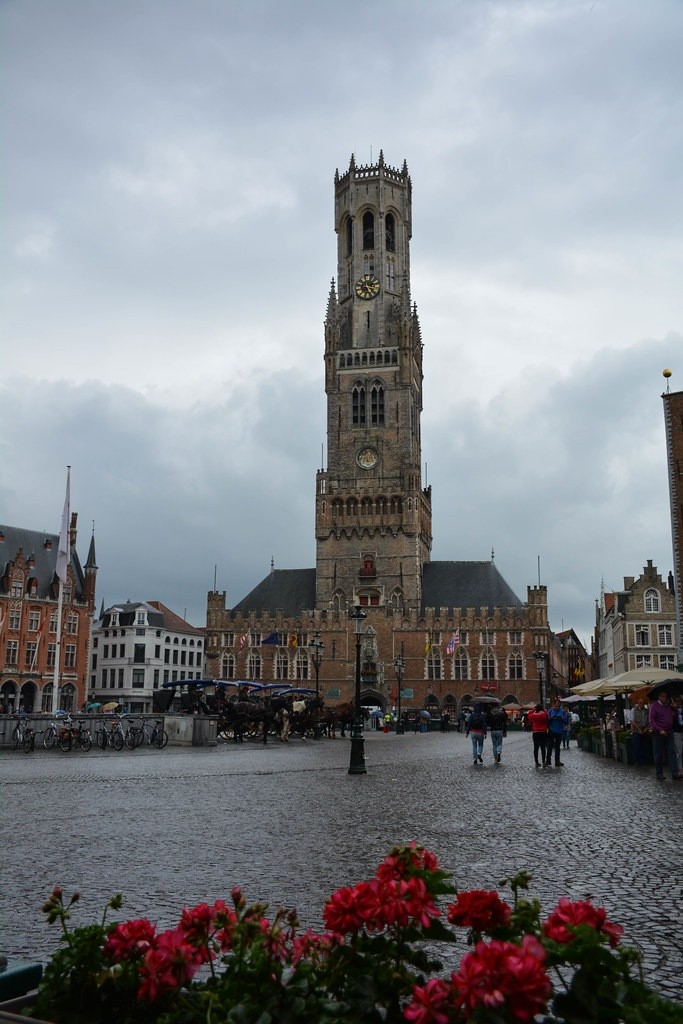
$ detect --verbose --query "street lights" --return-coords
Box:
[346,605,368,776]
[306,630,326,740]
[535,651,545,702]
[393,653,406,735]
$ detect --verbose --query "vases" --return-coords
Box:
[0,992,51,1024]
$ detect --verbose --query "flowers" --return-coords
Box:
[23,841,683,1023]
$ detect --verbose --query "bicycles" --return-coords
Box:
[10,715,34,754]
[133,716,168,749]
[42,714,93,753]
[96,716,141,751]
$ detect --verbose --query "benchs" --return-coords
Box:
[206,695,216,713]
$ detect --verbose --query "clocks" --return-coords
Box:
[356,275,380,300]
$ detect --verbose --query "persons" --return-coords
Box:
[401,709,470,733]
[378,713,390,726]
[184,685,252,715]
[522,713,531,732]
[607,693,683,780]
[88,704,128,713]
[527,700,579,766]
[466,707,508,764]
[0,703,32,714]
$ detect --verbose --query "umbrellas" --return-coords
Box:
[87,703,102,709]
[376,712,384,718]
[522,701,537,710]
[503,702,521,720]
[420,711,431,718]
[559,663,683,730]
[104,702,118,710]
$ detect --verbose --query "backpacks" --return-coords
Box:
[470,714,481,729]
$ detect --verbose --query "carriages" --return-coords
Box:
[150,676,371,746]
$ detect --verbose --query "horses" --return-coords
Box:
[210,696,370,744]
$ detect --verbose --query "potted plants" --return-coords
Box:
[577,726,632,764]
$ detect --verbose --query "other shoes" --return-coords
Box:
[546,762,551,765]
[543,764,547,767]
[672,775,683,781]
[474,761,477,764]
[657,774,666,780]
[536,763,541,768]
[497,752,501,762]
[556,762,564,766]
[478,755,483,762]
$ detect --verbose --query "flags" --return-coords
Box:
[240,632,278,649]
[426,630,459,655]
[56,474,71,583]
[34,620,45,638]
